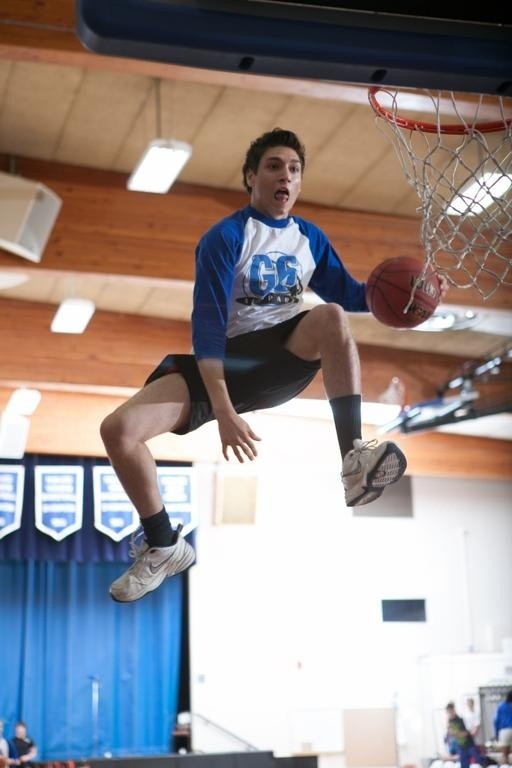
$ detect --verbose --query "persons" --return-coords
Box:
[445,691,512,763]
[0,718,14,762]
[100,129,448,601]
[10,724,36,762]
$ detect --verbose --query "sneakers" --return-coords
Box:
[109,523,196,602]
[341,439,406,506]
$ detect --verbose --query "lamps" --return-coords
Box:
[442,134,511,217]
[125,75,193,195]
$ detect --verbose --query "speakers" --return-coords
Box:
[0,169,63,264]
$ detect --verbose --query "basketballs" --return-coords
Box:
[364,256,442,328]
